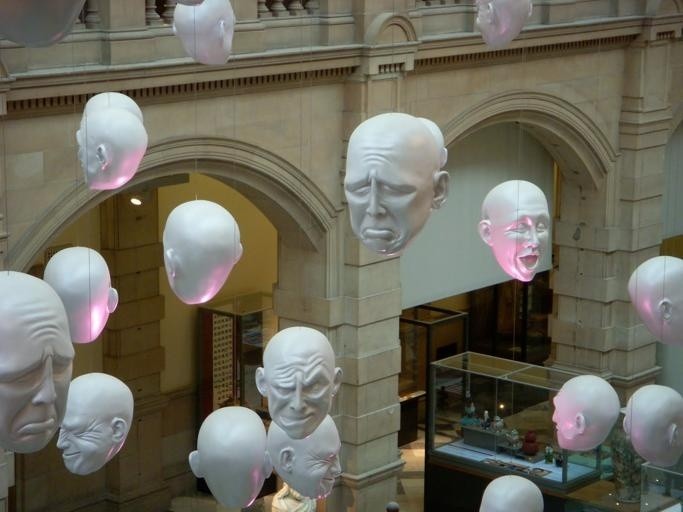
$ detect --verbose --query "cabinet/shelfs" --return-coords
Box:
[195,291,277,500]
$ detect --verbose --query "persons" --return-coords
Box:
[627,256,682,344]
[44,246,118,344]
[56,372,135,475]
[77,91,148,190]
[172,0,236,65]
[477,179,550,281]
[479,474,544,510]
[2,270,75,454]
[343,114,452,254]
[623,385,682,466]
[475,1,533,46]
[189,406,272,508]
[255,327,342,498]
[552,375,620,451]
[163,200,242,305]
